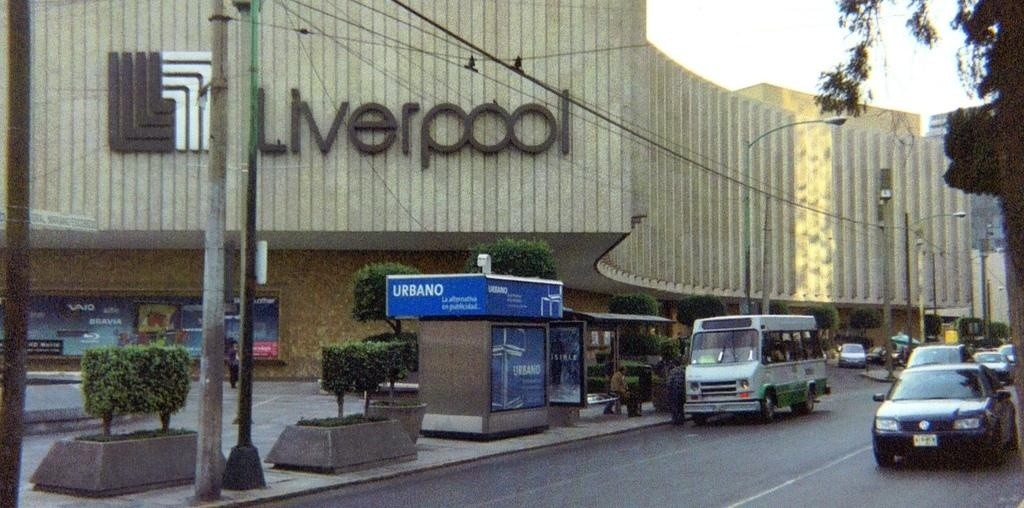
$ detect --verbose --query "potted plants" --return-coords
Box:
[28,345,227,497]
[652,338,688,413]
[263,262,427,473]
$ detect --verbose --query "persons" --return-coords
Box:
[601,351,616,416]
[665,356,685,425]
[611,367,642,418]
[227,339,238,387]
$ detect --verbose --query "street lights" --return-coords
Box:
[980,245,1004,338]
[902,210,965,361]
[735,114,848,313]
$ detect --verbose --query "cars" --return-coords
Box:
[871,363,1019,468]
[839,338,1016,386]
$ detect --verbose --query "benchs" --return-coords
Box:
[587,392,641,415]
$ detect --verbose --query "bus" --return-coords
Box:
[682,310,832,424]
